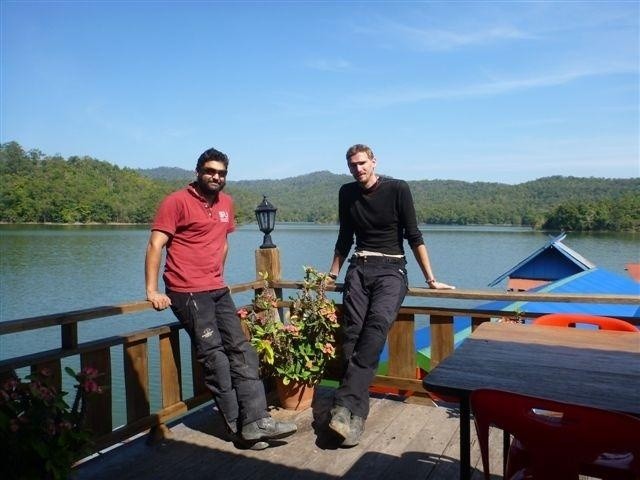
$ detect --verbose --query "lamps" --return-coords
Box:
[254,196,278,249]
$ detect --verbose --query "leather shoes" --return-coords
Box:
[232,417,297,450]
[326,401,366,447]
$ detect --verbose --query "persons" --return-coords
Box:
[143,145,299,451]
[322,143,458,448]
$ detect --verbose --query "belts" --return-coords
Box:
[352,257,405,264]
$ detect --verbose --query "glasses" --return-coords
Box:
[200,168,227,176]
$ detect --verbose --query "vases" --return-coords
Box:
[274,376,317,411]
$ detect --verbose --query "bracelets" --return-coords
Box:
[329,273,337,280]
[425,279,436,283]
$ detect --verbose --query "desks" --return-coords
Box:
[422,322,640,480]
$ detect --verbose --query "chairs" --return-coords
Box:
[504,312,640,480]
[469,388,640,480]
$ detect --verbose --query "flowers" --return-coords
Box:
[237,264,340,386]
[0,364,113,480]
